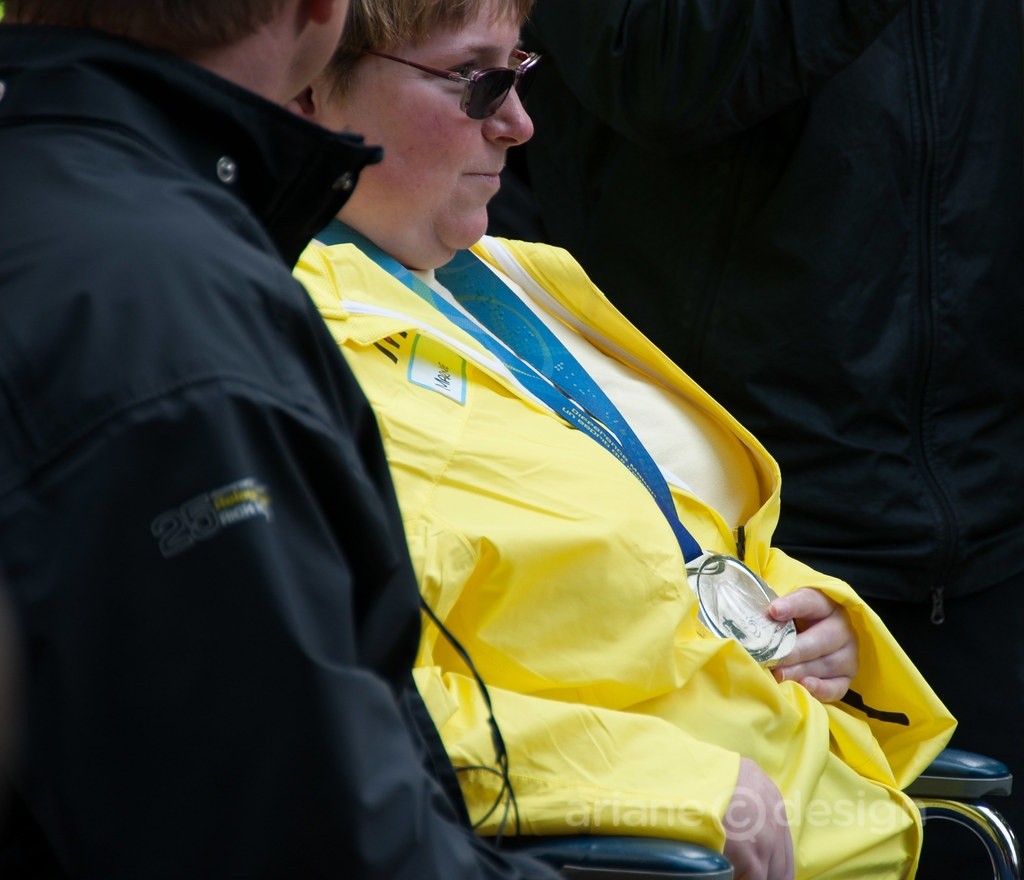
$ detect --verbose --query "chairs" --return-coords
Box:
[484,747,1024,880]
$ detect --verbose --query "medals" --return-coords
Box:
[685,552,797,664]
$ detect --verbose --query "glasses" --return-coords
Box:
[362,46,544,119]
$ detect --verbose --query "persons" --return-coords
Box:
[0,2,559,880]
[492,0,1022,880]
[287,0,959,880]
